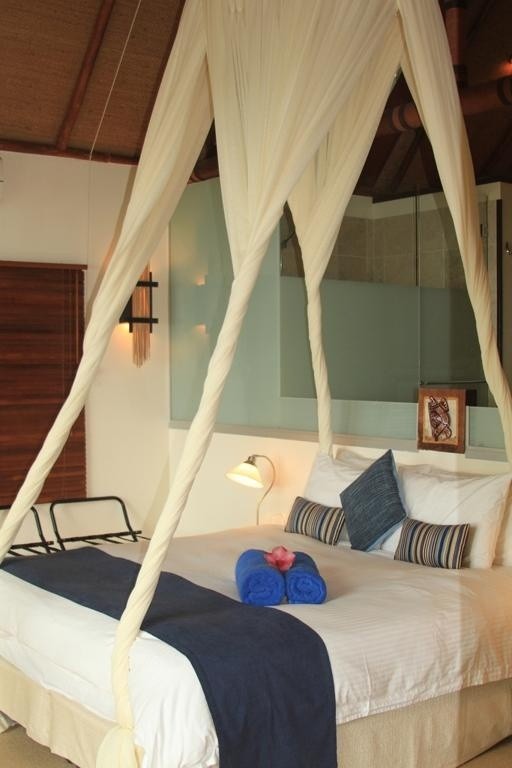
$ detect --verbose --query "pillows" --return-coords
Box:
[284,444,511,572]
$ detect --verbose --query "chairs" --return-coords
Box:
[0,496,142,562]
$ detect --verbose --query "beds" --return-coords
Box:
[0,445,512,768]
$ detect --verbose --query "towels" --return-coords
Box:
[234,547,326,607]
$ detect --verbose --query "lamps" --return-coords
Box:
[226,451,277,526]
[118,259,159,369]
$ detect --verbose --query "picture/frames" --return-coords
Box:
[415,386,466,454]
